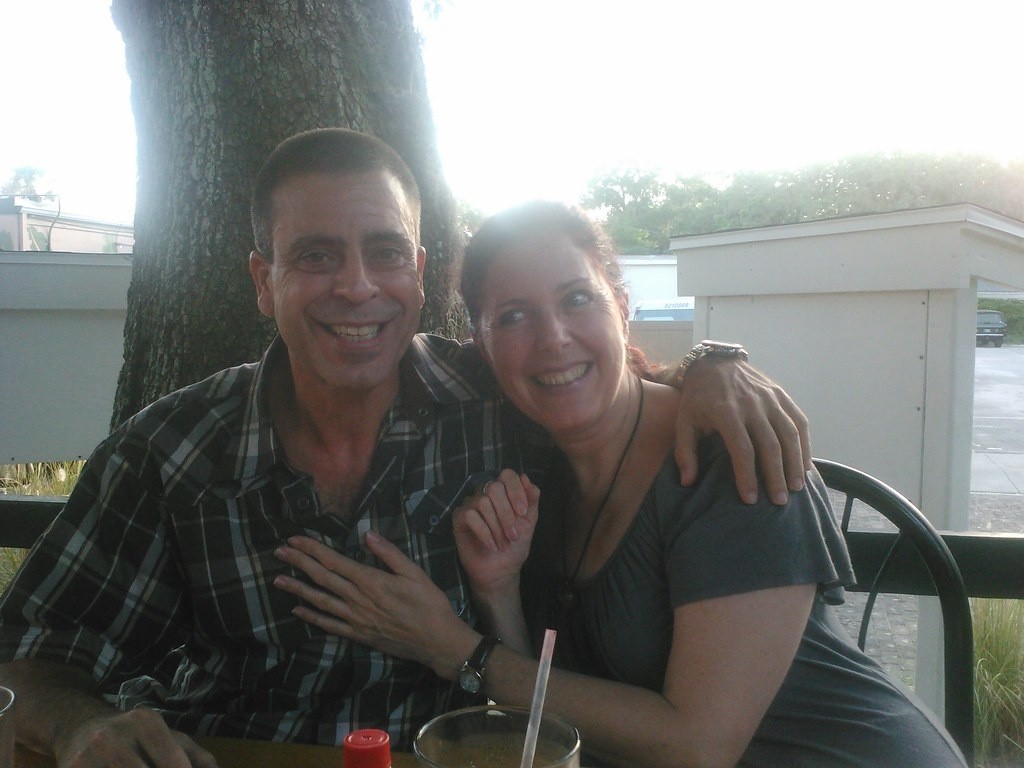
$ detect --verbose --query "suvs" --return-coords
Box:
[976,310,1008,348]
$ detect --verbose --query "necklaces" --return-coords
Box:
[560,374,643,593]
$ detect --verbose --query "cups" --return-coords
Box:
[414,705,581,768]
[0,685,16,768]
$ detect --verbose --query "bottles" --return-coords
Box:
[344,729,391,768]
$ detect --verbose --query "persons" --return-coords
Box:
[1,129,813,768]
[272,195,969,768]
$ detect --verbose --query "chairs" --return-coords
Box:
[812,458,974,768]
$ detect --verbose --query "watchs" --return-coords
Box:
[456,636,503,698]
[677,340,749,385]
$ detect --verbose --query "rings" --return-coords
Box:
[482,478,495,496]
